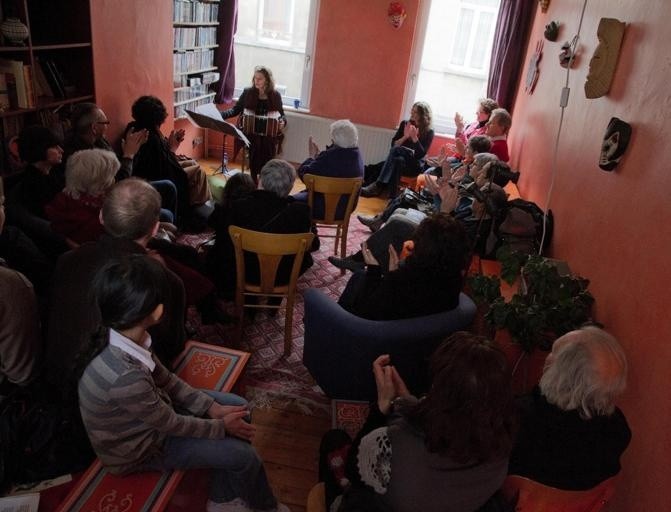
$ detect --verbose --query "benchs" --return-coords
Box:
[57,340,251,512]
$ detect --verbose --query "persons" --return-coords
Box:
[327,98,514,323]
[0,94,260,368]
[317,329,521,511]
[218,65,289,188]
[292,119,365,222]
[205,158,324,318]
[72,253,291,510]
[508,325,633,492]
[1,255,46,494]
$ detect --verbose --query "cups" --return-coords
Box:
[294,101,300,109]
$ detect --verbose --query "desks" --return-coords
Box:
[481,260,555,390]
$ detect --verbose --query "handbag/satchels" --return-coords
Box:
[402,185,431,207]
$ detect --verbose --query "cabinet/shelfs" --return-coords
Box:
[0,0,95,180]
[173,22,220,108]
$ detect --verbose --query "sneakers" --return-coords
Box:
[206,496,254,512]
[253,500,291,512]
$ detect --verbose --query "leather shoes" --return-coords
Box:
[328,255,365,272]
[184,326,200,339]
[361,181,383,196]
[356,214,382,232]
[202,312,239,327]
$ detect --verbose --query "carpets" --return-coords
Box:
[185,212,374,420]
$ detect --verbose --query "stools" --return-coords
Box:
[400,176,417,194]
[331,399,370,441]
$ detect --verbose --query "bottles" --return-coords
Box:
[1,17,29,47]
[223,152,229,173]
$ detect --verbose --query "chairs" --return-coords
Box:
[502,469,624,512]
[227,224,315,356]
[303,173,363,274]
[303,288,478,406]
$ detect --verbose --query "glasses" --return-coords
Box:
[98,121,111,125]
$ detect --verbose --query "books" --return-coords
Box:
[1,57,75,139]
[172,1,220,120]
